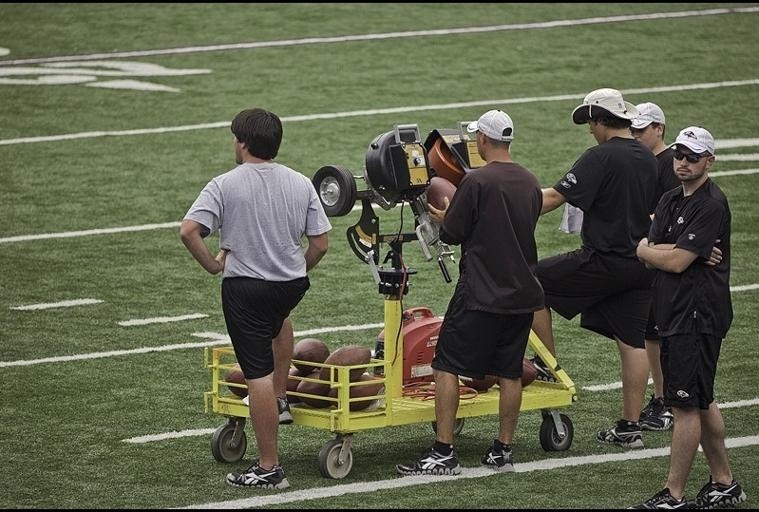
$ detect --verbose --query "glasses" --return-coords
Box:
[672,147,708,163]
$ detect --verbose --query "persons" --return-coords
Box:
[396,111,544,475]
[629,101,675,432]
[179,107,332,490]
[528,89,660,448]
[626,125,747,509]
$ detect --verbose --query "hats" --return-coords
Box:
[572,89,667,129]
[468,111,514,142]
[674,128,714,155]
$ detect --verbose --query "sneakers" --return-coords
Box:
[481,444,516,471]
[687,479,748,509]
[529,358,562,385]
[397,448,460,474]
[227,464,290,491]
[598,419,644,451]
[640,397,674,432]
[275,397,292,424]
[627,485,687,511]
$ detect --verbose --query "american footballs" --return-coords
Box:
[458,356,539,389]
[427,177,456,211]
[226,338,385,410]
